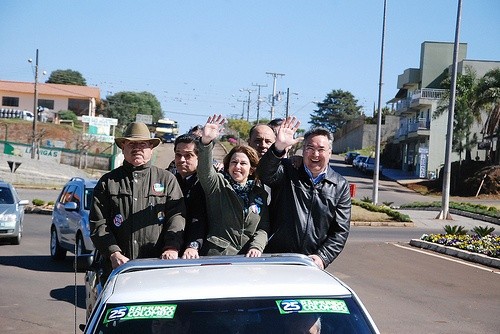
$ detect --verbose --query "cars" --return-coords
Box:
[344,152,376,179]
[79,253,381,334]
[0,180,30,246]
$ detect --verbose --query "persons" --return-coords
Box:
[256,115,351,270]
[166,125,225,177]
[174,134,209,259]
[246,119,293,208]
[285,313,321,334]
[198,114,274,256]
[89,122,188,275]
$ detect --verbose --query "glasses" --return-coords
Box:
[304,147,331,155]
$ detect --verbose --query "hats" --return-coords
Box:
[114,122,161,149]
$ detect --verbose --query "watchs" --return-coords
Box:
[190,242,199,249]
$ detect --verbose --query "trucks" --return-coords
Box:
[146,118,179,143]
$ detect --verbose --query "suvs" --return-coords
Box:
[50,178,99,261]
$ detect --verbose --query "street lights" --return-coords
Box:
[27,48,46,158]
[285,87,319,159]
[257,92,299,121]
